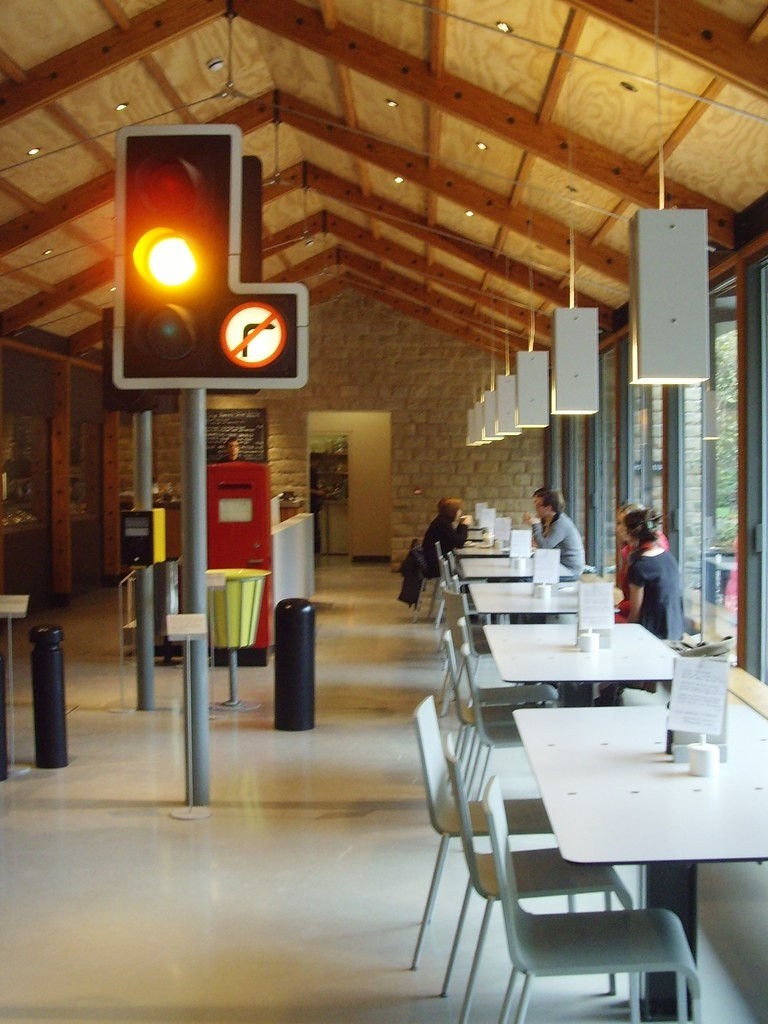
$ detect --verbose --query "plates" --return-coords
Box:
[464,543,477,547]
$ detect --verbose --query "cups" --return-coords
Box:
[494,540,504,550]
[580,633,600,653]
[483,533,494,547]
[688,744,720,777]
[535,585,551,600]
[511,559,527,572]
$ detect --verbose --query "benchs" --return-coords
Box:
[578,570,767,721]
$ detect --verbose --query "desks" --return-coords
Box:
[483,623,684,707]
[459,558,574,583]
[511,706,768,1024]
[450,520,512,556]
[468,580,621,625]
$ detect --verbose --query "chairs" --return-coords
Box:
[408,538,701,1024]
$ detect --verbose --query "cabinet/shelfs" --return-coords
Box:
[311,452,350,555]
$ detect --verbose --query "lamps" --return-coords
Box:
[467,1,711,446]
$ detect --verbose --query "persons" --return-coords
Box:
[309,452,326,555]
[523,488,585,625]
[595,503,685,706]
[422,498,472,578]
[216,437,246,463]
[2,443,30,501]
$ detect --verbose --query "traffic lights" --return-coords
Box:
[113,127,228,389]
